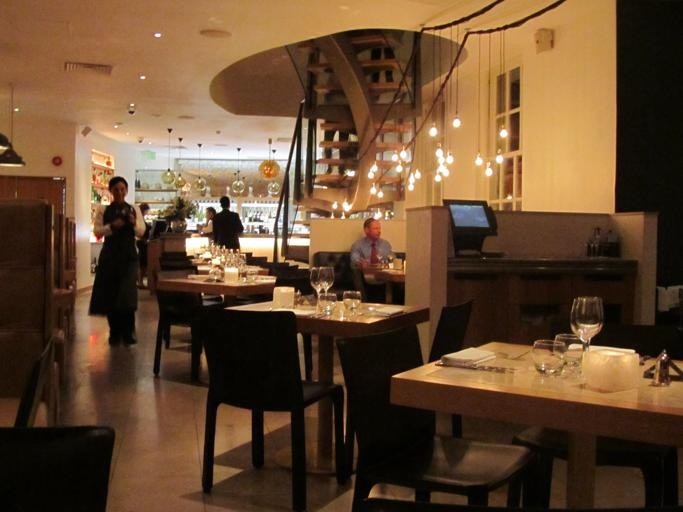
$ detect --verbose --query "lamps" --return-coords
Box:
[0,79,28,167]
[162,128,281,197]
[429,25,508,177]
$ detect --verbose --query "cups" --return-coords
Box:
[208,241,247,284]
[318,292,337,316]
[553,333,588,374]
[342,289,361,317]
[529,339,567,375]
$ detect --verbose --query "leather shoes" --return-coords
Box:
[125,332,137,344]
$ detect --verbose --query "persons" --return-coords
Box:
[349,218,390,271]
[195,206,216,235]
[212,196,244,250]
[86,176,146,345]
[135,202,151,289]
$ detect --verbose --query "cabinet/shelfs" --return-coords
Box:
[135,188,176,205]
[91,149,114,207]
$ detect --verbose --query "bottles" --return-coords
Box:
[585,226,619,256]
[247,210,264,222]
[654,352,670,386]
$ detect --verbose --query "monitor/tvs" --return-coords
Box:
[443,200,505,256]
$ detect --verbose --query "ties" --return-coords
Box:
[371,245,377,265]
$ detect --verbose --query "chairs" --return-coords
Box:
[0,427,116,512]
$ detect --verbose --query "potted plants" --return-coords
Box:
[158,197,197,232]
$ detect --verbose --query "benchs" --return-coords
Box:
[0,200,73,402]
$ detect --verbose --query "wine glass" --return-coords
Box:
[306,265,335,314]
[569,295,606,382]
[377,251,393,268]
